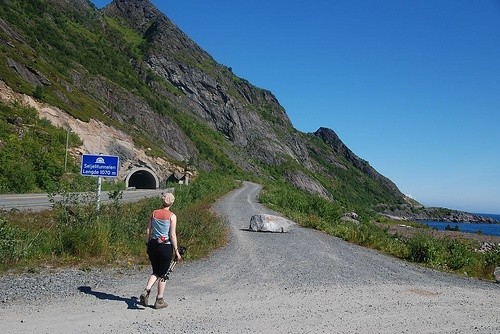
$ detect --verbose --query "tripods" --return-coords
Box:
[160,246,187,283]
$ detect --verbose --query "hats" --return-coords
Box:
[162,193,175,206]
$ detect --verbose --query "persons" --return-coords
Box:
[140,193,182,308]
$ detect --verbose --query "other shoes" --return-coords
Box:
[154,300,168,308]
[140,291,148,307]
